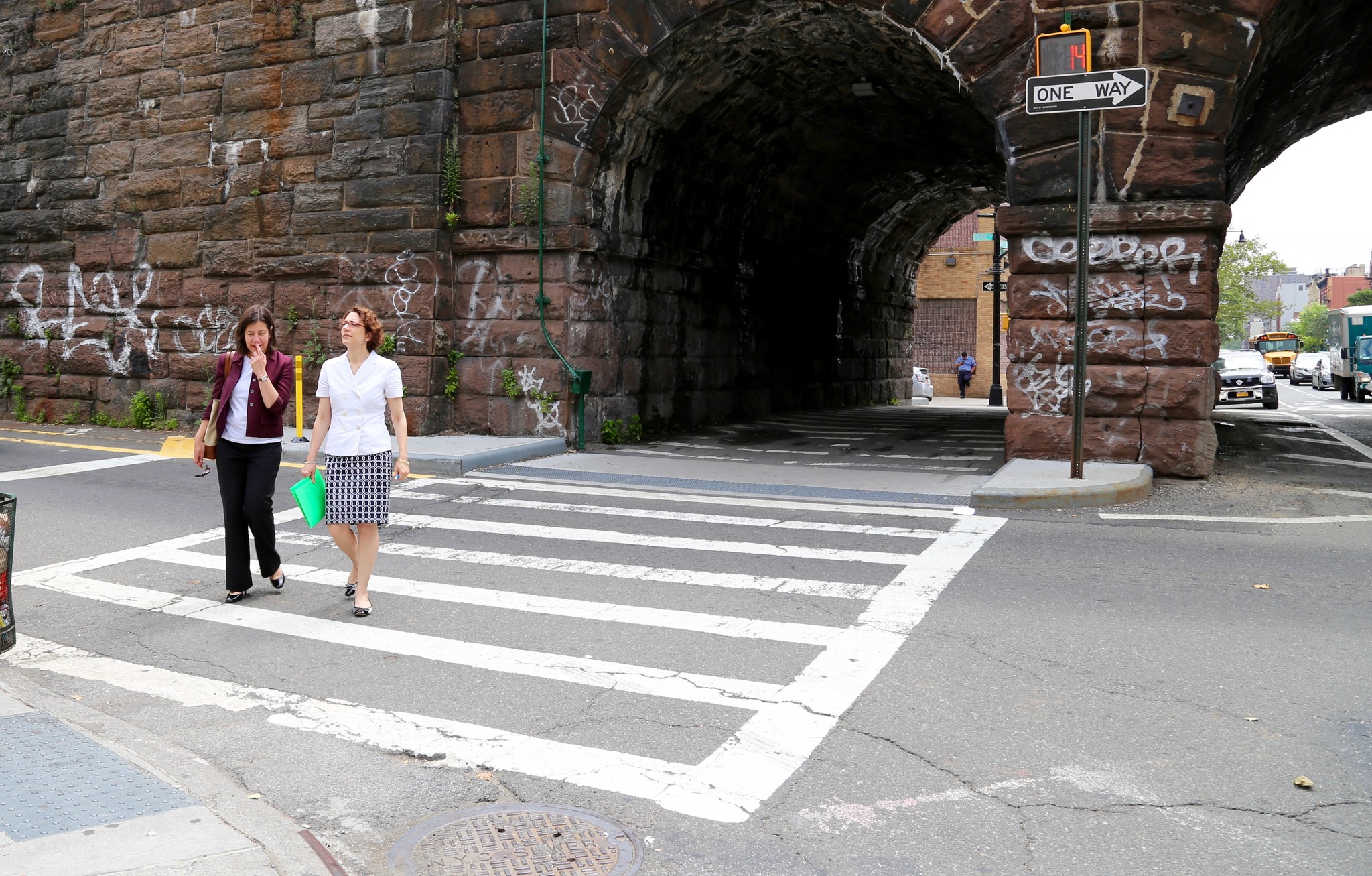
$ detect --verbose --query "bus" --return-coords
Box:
[1247,331,1304,377]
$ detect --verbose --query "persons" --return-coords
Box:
[191,306,294,603]
[301,307,410,617]
[955,352,977,399]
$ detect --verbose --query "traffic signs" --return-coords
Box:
[982,281,1008,292]
[1024,65,1149,117]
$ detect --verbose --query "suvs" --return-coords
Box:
[1287,352,1327,386]
[1211,348,1279,411]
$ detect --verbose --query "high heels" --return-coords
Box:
[226,589,247,603]
[270,573,285,589]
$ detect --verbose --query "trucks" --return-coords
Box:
[1326,304,1372,404]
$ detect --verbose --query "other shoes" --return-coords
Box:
[353,597,373,616]
[966,382,971,387]
[343,577,358,596]
[960,394,964,399]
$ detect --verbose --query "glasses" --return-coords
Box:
[338,321,370,332]
[194,461,211,478]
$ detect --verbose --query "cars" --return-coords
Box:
[912,364,934,402]
[1311,355,1334,391]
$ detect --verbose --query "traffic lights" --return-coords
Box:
[1036,27,1092,74]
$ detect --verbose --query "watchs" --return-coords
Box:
[256,374,269,382]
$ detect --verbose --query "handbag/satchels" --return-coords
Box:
[202,350,237,460]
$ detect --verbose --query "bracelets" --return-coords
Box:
[304,461,317,464]
[397,458,410,466]
